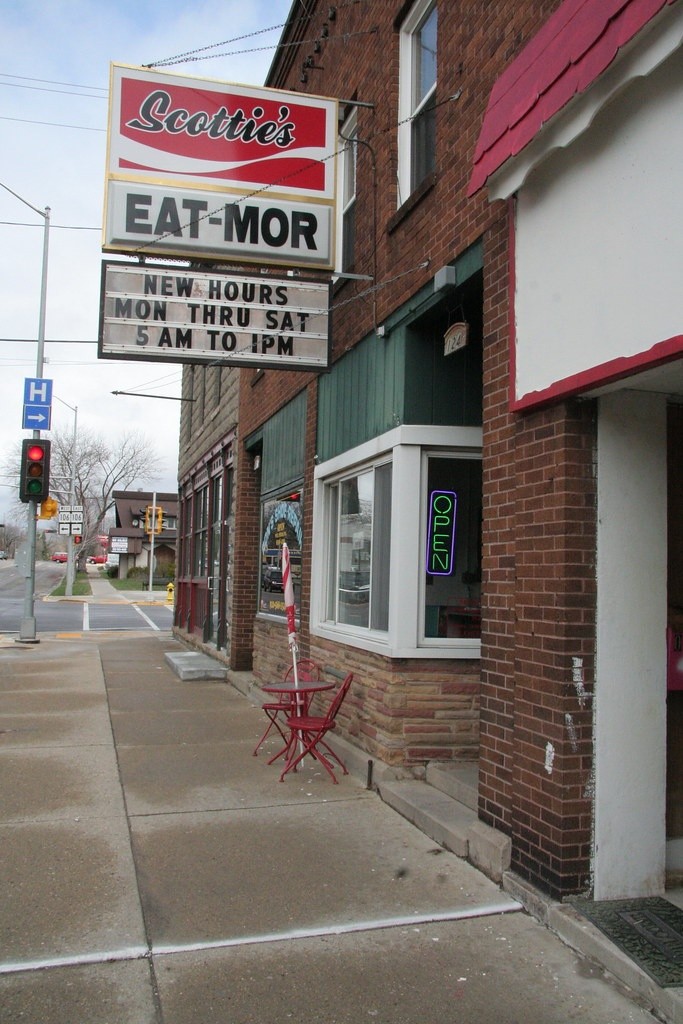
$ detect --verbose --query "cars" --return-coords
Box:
[0,551,8,560]
[90,554,107,564]
[263,567,296,594]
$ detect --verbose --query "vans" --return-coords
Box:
[52,551,67,563]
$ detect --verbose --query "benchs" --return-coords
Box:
[142,581,169,591]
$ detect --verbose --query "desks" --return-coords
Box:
[260,682,335,767]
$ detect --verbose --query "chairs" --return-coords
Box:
[278,674,352,783]
[253,658,318,756]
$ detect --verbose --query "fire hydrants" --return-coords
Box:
[165,582,174,603]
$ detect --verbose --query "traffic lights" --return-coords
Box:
[139,505,149,532]
[158,507,168,534]
[75,536,82,544]
[275,491,301,503]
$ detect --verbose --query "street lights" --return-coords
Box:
[19,439,52,504]
